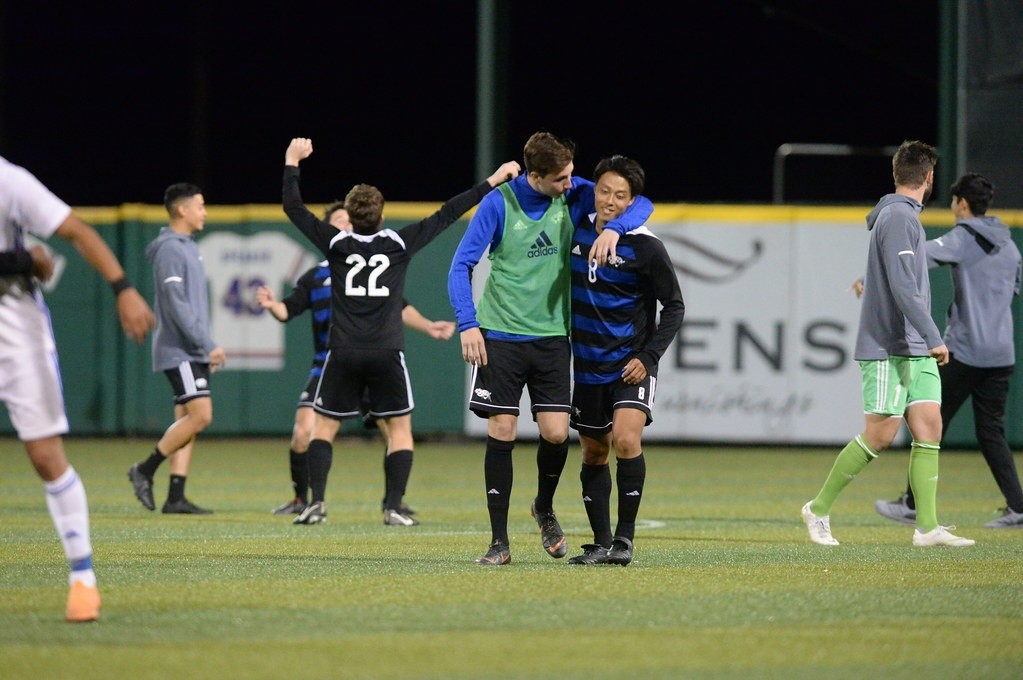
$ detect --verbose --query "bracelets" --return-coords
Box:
[110,276,134,295]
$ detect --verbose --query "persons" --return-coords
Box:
[874,171,1022,527]
[569,155,686,568]
[125,183,226,514]
[447,132,654,566]
[281,134,520,528]
[801,141,978,547]
[0,150,156,621]
[257,203,457,517]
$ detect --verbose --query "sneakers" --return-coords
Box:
[913,525,975,547]
[475,538,511,565]
[162,499,212,515]
[801,499,840,546]
[531,496,568,558]
[128,463,155,511]
[875,492,917,526]
[293,501,327,524]
[383,508,419,526]
[382,501,415,515]
[569,544,613,564]
[607,536,633,566]
[65,578,101,621]
[985,505,1023,529]
[271,500,308,515]
